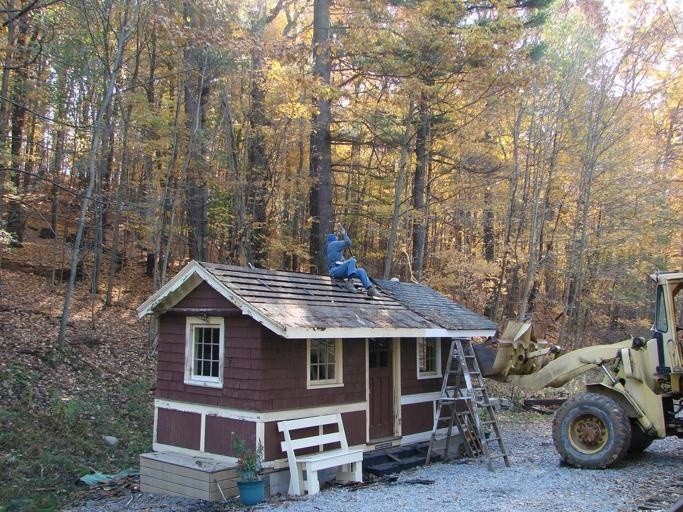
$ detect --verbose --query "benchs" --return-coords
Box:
[277,413,365,496]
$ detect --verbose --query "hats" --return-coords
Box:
[327,233,337,241]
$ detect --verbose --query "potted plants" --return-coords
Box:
[232,437,265,506]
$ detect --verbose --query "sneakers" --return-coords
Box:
[343,280,363,295]
[366,286,385,298]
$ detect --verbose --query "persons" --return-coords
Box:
[323,224,381,296]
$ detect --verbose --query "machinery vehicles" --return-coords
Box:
[462,266,683,475]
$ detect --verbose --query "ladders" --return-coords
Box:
[425,338,510,471]
